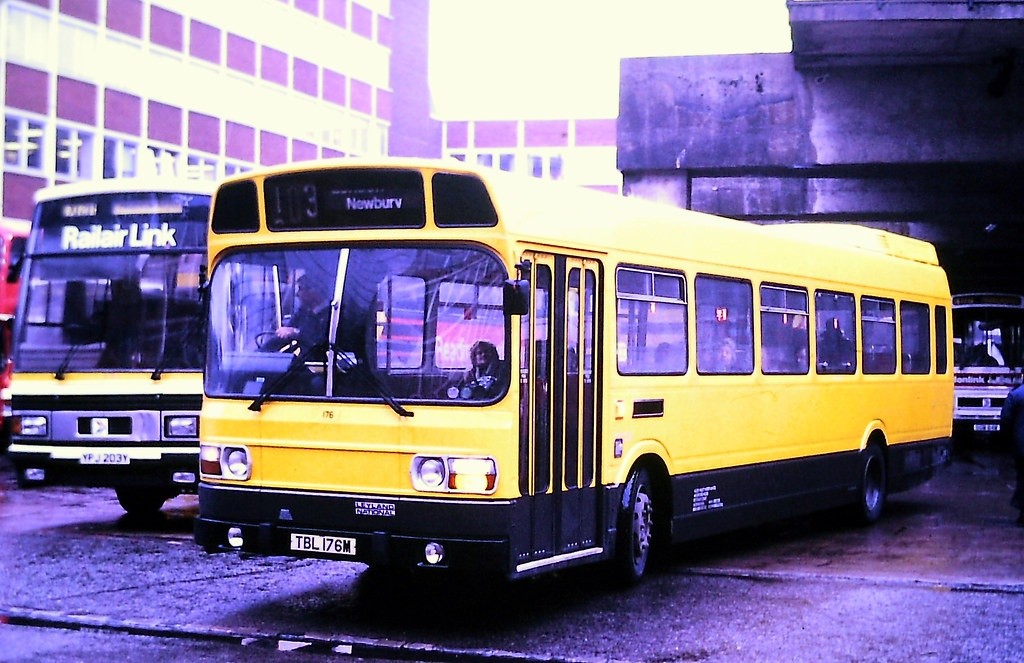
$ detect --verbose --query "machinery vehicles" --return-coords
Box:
[182,154,957,584]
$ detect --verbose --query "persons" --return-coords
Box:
[655,342,677,363]
[275,273,341,396]
[717,337,737,370]
[1001,353,1024,527]
[464,340,506,399]
[794,345,819,371]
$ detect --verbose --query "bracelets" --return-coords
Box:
[294,328,300,338]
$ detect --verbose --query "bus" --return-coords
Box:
[943,292,1024,436]
[1,180,506,533]
